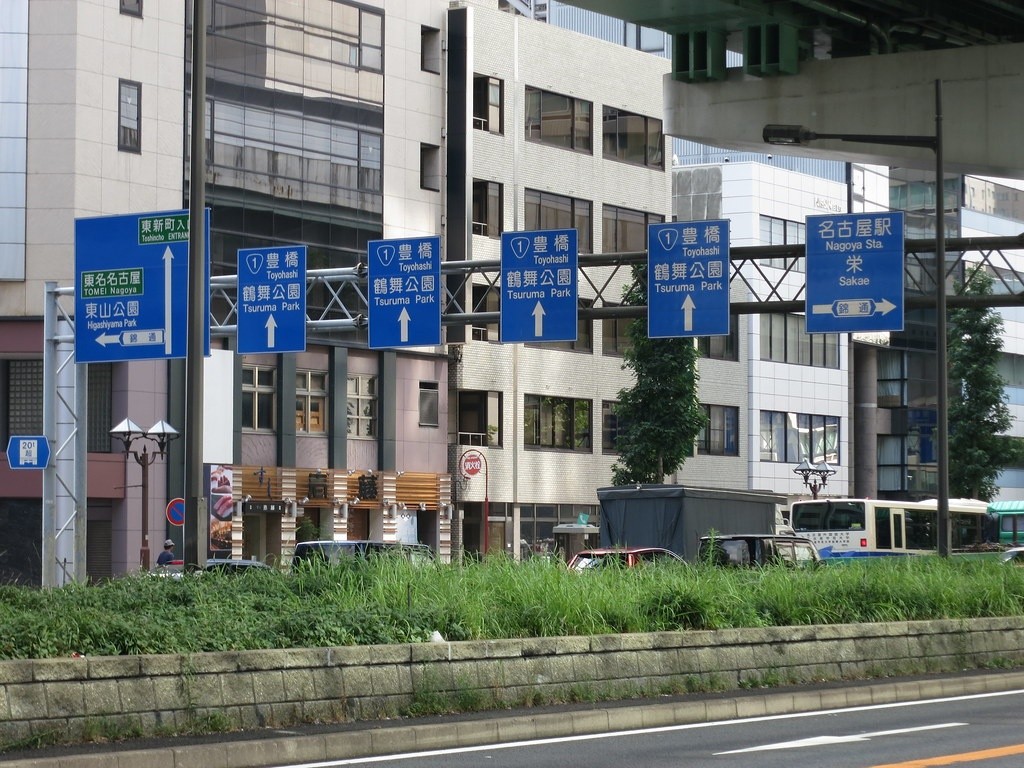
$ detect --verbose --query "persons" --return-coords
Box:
[156,539,175,567]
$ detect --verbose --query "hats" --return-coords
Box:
[164,539,175,547]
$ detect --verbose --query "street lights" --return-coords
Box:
[106,414,181,578]
[761,75,951,560]
[792,459,839,501]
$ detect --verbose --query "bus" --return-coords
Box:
[788,495,1000,564]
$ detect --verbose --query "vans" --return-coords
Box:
[291,541,434,577]
[699,533,825,571]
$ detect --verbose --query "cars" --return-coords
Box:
[566,546,689,579]
[206,559,276,574]
[997,548,1024,564]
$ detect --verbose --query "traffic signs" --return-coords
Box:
[646,217,731,340]
[499,228,579,344]
[235,244,310,355]
[802,210,907,333]
[72,210,192,364]
[367,236,441,350]
